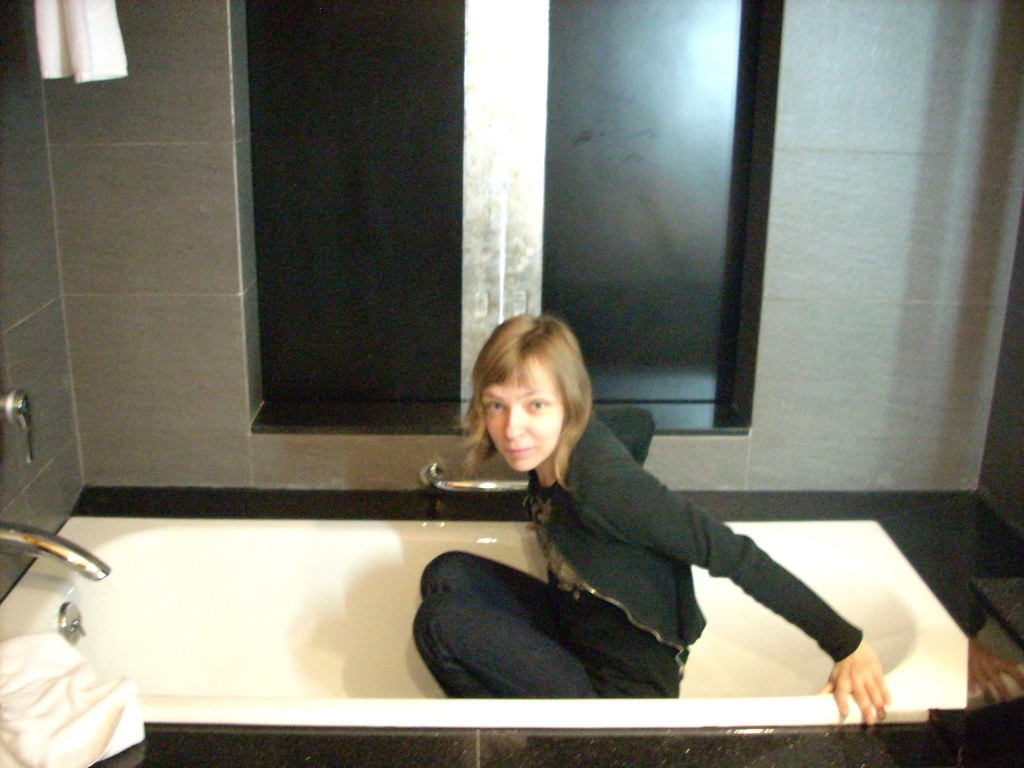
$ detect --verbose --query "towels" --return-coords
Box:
[0,630,152,768]
[33,0,130,86]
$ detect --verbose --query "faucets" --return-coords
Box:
[0,518,113,585]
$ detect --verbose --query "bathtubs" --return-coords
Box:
[2,512,973,735]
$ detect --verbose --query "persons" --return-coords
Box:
[412,316,889,726]
